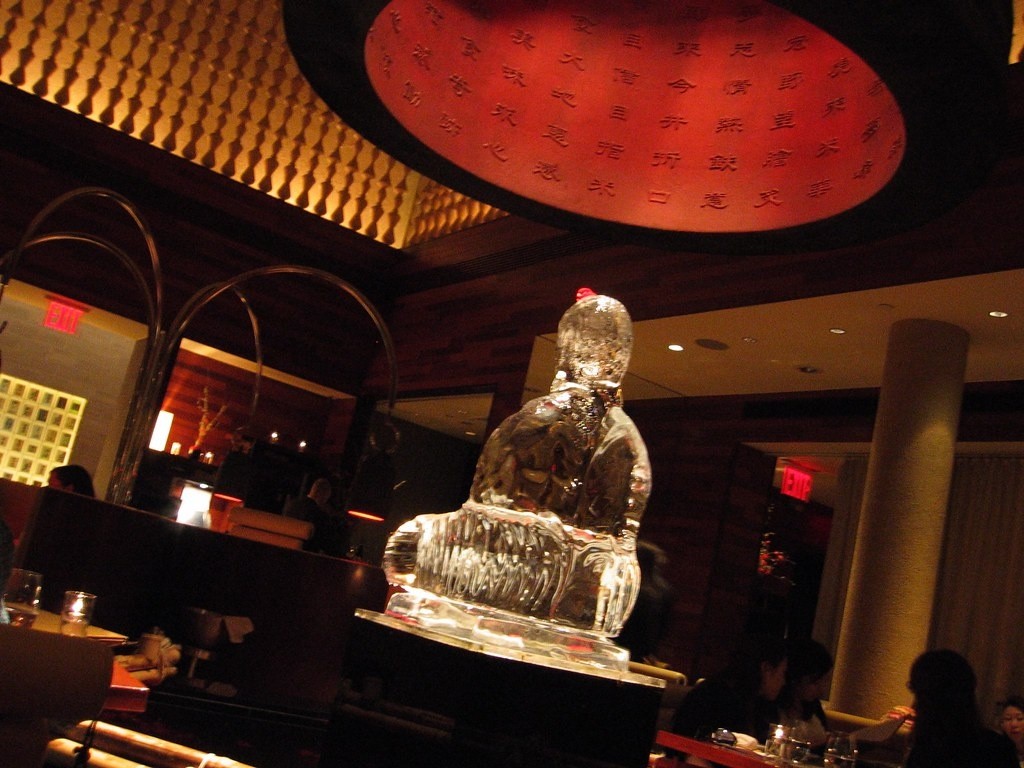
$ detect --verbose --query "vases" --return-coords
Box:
[188,446,205,461]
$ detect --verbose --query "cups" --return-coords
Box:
[61,589,96,635]
[767,724,858,768]
[3,568,43,625]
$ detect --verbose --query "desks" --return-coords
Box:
[102,659,150,713]
[0,601,129,646]
[655,730,793,768]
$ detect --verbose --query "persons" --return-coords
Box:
[0,489,16,603]
[233,435,255,452]
[611,542,668,663]
[48,464,95,498]
[294,480,348,555]
[994,696,1024,768]
[662,637,834,768]
[903,649,1021,768]
